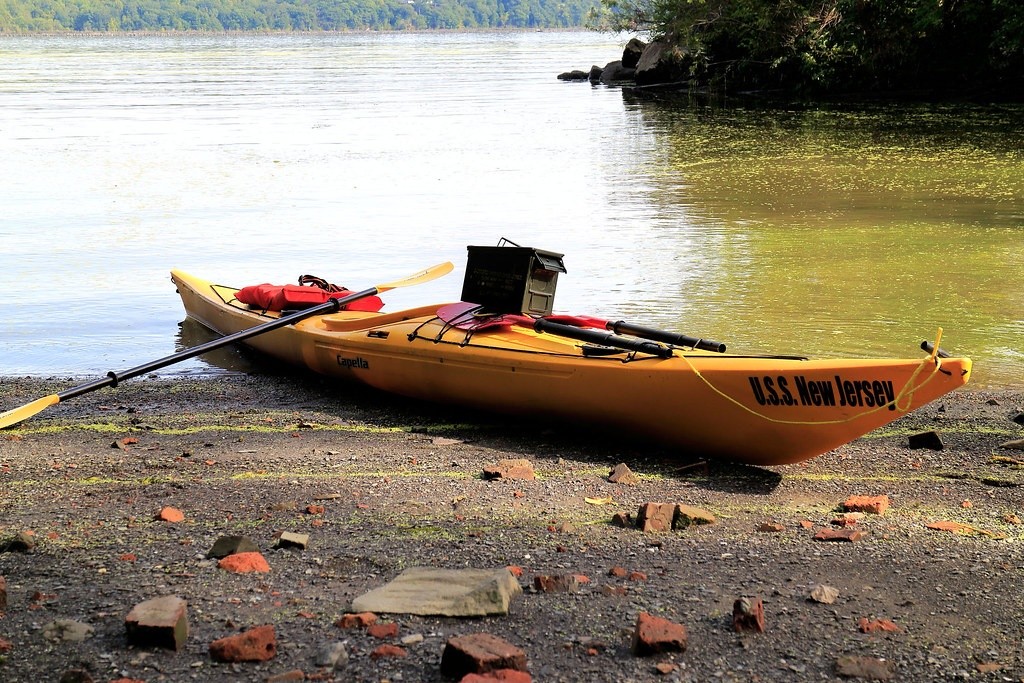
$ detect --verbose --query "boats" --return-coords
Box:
[171,269,973,466]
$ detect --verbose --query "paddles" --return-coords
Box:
[0,259,454,430]
[541,314,727,353]
[436,302,672,358]
